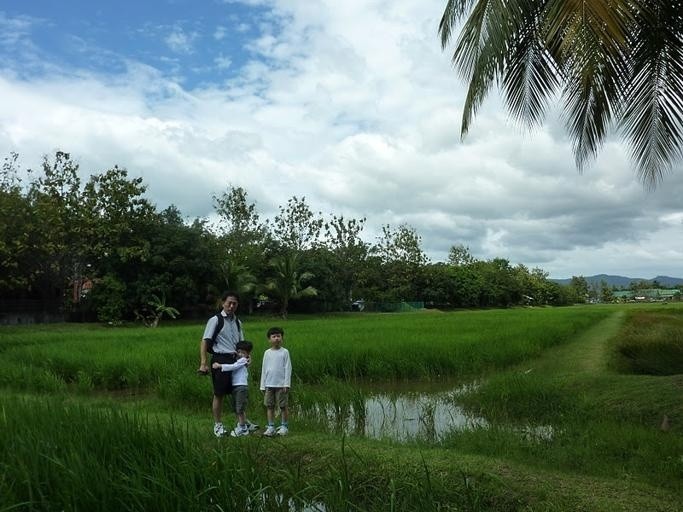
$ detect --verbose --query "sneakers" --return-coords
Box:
[263,424,276,437]
[230,419,259,438]
[277,425,289,436]
[214,423,228,437]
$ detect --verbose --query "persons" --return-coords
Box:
[212,341,253,437]
[200,291,259,438]
[259,327,292,437]
